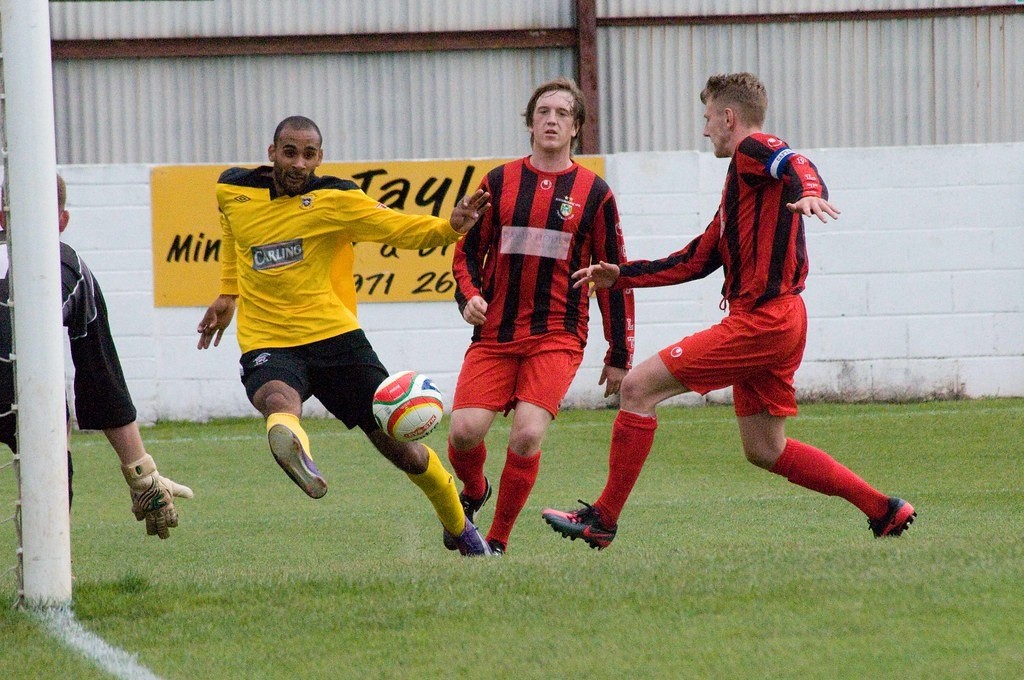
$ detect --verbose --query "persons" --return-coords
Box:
[0,174,194,609]
[197,117,492,559]
[446,77,635,557]
[545,73,917,550]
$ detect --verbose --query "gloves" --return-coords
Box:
[121,455,194,539]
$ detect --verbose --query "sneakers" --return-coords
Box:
[867,497,918,537]
[444,516,491,556]
[443,476,492,550]
[540,500,618,550]
[486,540,507,557]
[268,424,328,499]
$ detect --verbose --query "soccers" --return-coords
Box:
[372,368,445,444]
